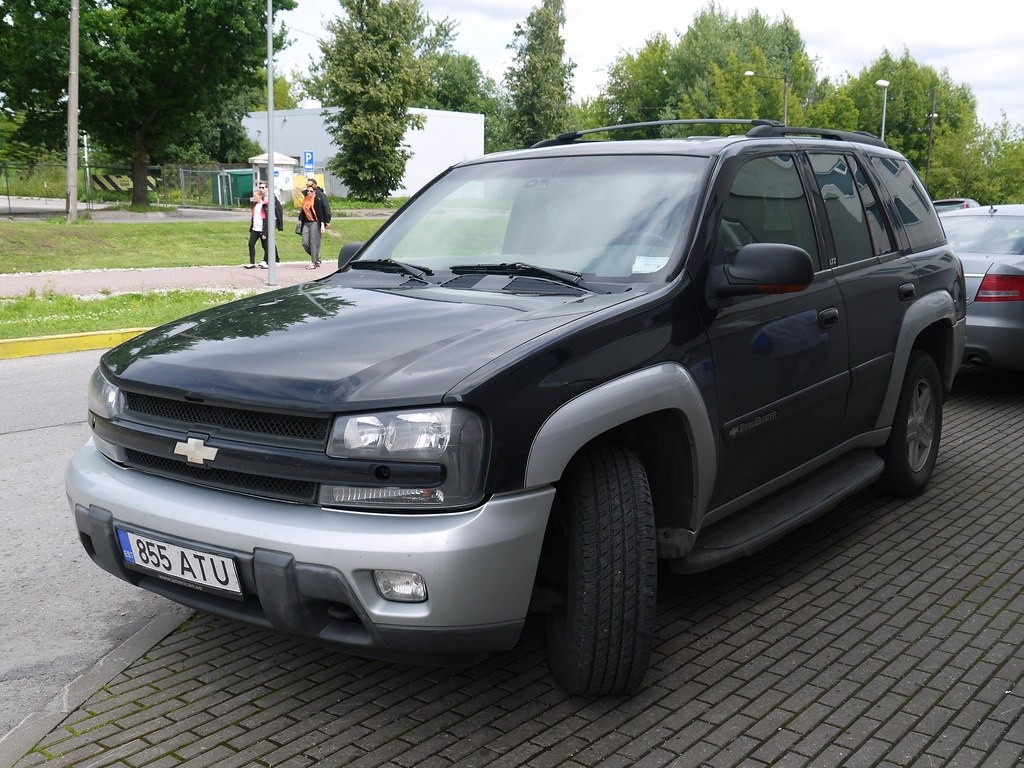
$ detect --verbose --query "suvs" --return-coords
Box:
[65,121,968,700]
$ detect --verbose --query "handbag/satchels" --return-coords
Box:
[295,224,300,236]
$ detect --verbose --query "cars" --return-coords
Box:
[938,205,1024,372]
[931,197,979,214]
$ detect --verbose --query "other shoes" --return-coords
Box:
[243,264,256,270]
[259,261,268,270]
[305,259,322,270]
[276,262,280,266]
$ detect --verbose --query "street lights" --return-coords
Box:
[742,71,787,126]
[875,79,891,140]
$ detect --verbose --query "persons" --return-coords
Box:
[259,181,283,266]
[298,186,325,270]
[306,179,332,263]
[244,190,269,269]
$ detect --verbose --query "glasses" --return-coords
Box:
[306,191,312,194]
[258,187,264,191]
